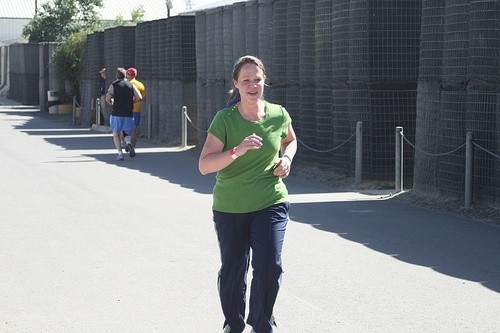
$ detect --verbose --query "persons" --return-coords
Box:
[99,68,106,97]
[198,55,298,333]
[105,68,147,160]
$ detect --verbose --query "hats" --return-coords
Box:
[127,68,137,76]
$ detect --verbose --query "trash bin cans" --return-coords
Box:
[46,88,74,116]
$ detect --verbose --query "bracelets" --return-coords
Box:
[284,155,291,163]
[230,146,239,159]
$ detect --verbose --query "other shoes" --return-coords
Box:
[116,153,124,160]
[127,144,136,158]
